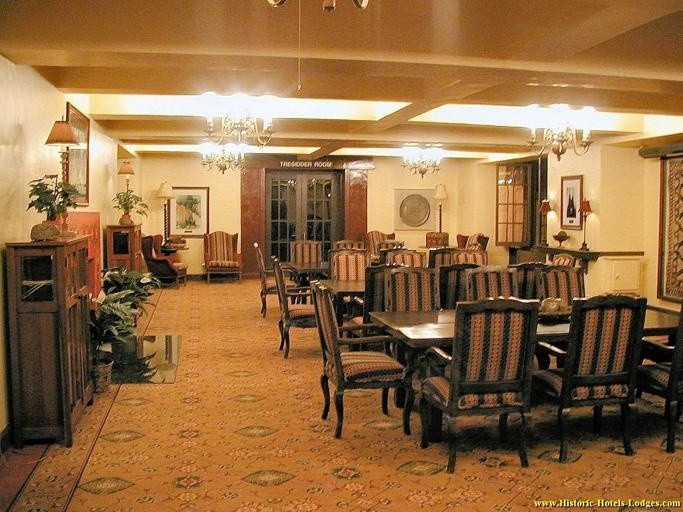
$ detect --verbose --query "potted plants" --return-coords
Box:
[26,173,86,239]
[89,265,157,394]
[111,190,148,225]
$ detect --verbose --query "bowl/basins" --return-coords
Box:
[539,311,572,322]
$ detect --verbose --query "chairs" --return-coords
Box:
[153,234,181,262]
[140,235,187,290]
[204,231,242,284]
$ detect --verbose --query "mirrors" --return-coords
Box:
[65,101,90,206]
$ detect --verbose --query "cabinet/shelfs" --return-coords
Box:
[4,233,93,448]
[105,224,143,273]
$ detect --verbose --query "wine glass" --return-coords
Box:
[553,235,570,248]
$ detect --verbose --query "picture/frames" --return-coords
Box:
[166,186,209,237]
[560,174,583,230]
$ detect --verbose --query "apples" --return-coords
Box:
[555,298,569,311]
[541,297,559,312]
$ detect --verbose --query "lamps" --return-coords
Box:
[117,159,135,192]
[400,146,443,180]
[266,0,368,11]
[578,197,591,251]
[155,181,175,240]
[197,95,275,174]
[45,119,80,238]
[431,183,448,232]
[538,200,552,246]
[524,104,599,160]
